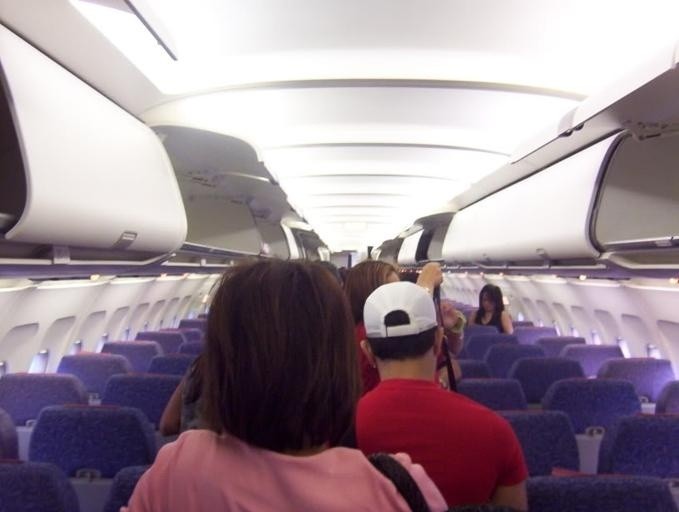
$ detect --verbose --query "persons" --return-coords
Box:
[352,282,528,511]
[316,260,518,398]
[156,353,221,435]
[114,258,448,512]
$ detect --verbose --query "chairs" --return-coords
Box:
[2,299,218,511]
[421,294,679,511]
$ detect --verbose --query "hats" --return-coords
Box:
[364,281,438,338]
